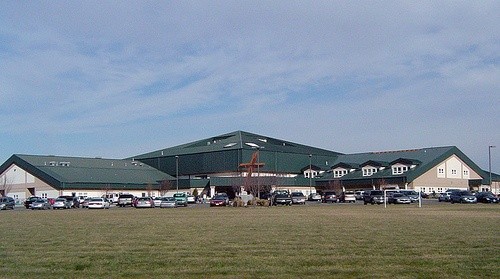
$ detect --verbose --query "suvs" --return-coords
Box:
[272,190,291,206]
[174,193,188,206]
[363,189,388,205]
[450,190,478,204]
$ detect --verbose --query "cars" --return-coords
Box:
[386,191,398,204]
[87,198,111,209]
[308,192,322,201]
[160,197,178,208]
[401,190,428,203]
[475,191,500,204]
[187,195,196,203]
[209,193,230,207]
[291,192,306,204]
[355,191,364,200]
[338,191,356,203]
[322,191,338,203]
[26,192,162,210]
[0,196,15,210]
[392,193,412,204]
[256,192,274,206]
[438,192,451,202]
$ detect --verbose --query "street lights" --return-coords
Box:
[488,145,496,193]
[308,154,312,195]
[175,156,180,193]
[257,146,261,198]
